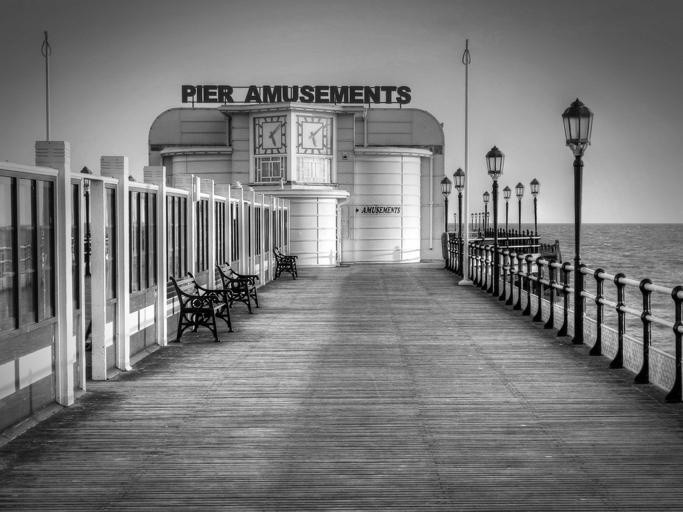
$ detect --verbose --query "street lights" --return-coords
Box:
[453,167,465,275]
[503,178,540,254]
[560,96,595,342]
[483,190,491,239]
[440,176,452,270]
[485,144,505,297]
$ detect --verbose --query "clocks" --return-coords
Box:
[253,114,287,156]
[295,114,333,156]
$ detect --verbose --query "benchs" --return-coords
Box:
[169,262,259,343]
[273,246,298,281]
